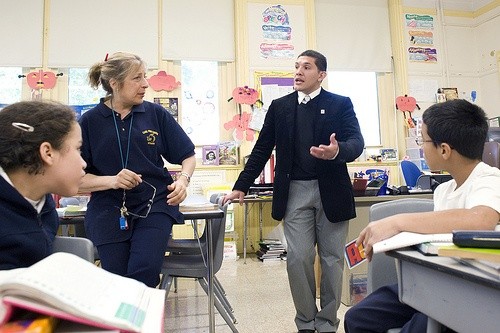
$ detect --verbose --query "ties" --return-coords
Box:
[305,96,310,102]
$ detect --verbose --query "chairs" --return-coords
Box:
[54,235,94,264]
[368,197,433,295]
[158,193,240,333]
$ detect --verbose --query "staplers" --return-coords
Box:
[366,169,385,181]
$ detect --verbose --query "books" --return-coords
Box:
[371,231,500,279]
[256,241,286,262]
[0,251,166,333]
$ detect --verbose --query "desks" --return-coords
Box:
[314,194,433,306]
[385,247,500,333]
[57,194,225,333]
[234,194,280,259]
[231,198,273,264]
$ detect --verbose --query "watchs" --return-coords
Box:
[179,173,190,183]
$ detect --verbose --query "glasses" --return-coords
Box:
[416,136,453,151]
[114,180,157,219]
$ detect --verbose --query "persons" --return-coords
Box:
[220,50,365,333]
[344,98,500,333]
[206,151,217,162]
[76,50,197,287]
[0,99,87,271]
